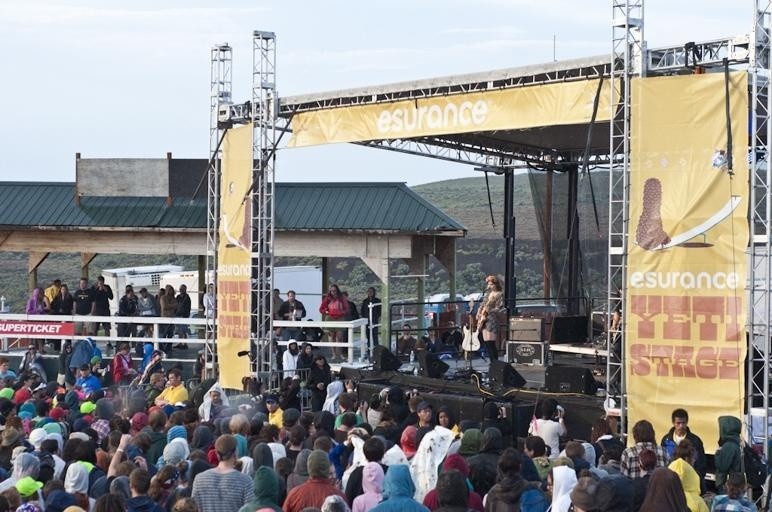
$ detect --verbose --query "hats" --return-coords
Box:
[570,478,611,512]
[48,408,69,420]
[44,489,76,512]
[16,477,44,497]
[80,364,89,371]
[369,394,382,410]
[415,402,433,411]
[3,427,22,446]
[540,400,558,416]
[139,288,147,293]
[265,395,277,403]
[22,374,36,382]
[163,437,190,465]
[15,503,41,512]
[81,402,95,413]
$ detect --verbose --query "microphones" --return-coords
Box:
[481,287,491,292]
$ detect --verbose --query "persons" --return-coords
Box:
[476,275,503,362]
[203,284,213,338]
[343,389,552,512]
[1,341,368,512]
[395,320,464,363]
[547,408,757,512]
[528,398,566,457]
[361,287,381,348]
[116,284,191,357]
[26,277,113,355]
[267,288,324,342]
[610,286,623,381]
[320,284,359,361]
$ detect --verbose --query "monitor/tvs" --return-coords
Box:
[549,316,590,344]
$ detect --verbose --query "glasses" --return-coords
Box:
[27,347,36,350]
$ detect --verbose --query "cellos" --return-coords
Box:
[462,298,480,353]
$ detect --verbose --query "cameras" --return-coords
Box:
[295,378,302,385]
[343,380,357,392]
[497,404,503,416]
[554,406,563,418]
[405,389,419,398]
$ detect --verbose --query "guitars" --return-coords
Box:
[476,292,503,331]
